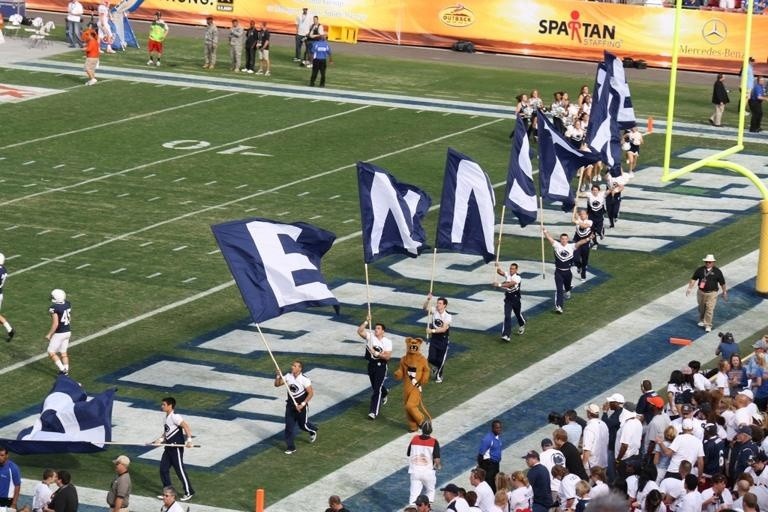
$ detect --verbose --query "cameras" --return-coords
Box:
[718,332,726,342]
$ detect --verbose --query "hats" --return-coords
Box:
[681,405,692,414]
[112,455,130,465]
[440,484,459,492]
[702,254,716,262]
[541,438,553,447]
[737,389,754,400]
[584,403,599,415]
[606,393,626,403]
[521,450,539,458]
[682,418,693,430]
[752,340,768,350]
[413,495,429,503]
[422,420,433,435]
[734,424,753,436]
[646,396,664,408]
[701,423,717,432]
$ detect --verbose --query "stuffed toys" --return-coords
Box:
[394,337,433,433]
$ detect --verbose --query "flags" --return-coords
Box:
[210,49,636,325]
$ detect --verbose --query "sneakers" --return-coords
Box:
[382,388,389,405]
[310,432,317,443]
[5,328,16,342]
[705,327,711,332]
[565,291,571,299]
[179,495,193,501]
[434,376,444,383]
[501,335,510,342]
[517,320,527,335]
[555,305,563,313]
[368,412,376,419]
[697,322,704,326]
[156,495,163,499]
[284,449,297,454]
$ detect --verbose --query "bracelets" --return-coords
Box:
[158,436,165,442]
[184,434,192,444]
[276,374,280,378]
[374,351,379,357]
[300,401,306,407]
[363,320,368,325]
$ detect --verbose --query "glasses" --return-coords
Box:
[705,262,711,264]
[416,503,421,506]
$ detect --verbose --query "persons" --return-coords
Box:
[0,444,187,512]
[399,254,768,512]
[708,56,768,132]
[275,360,318,454]
[0,254,15,342]
[44,287,73,373]
[63,0,333,88]
[515,86,643,314]
[153,398,195,502]
[325,493,344,511]
[357,316,393,418]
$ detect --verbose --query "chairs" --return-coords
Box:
[4,13,57,50]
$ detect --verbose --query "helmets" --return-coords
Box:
[0,252,5,266]
[48,289,67,305]
